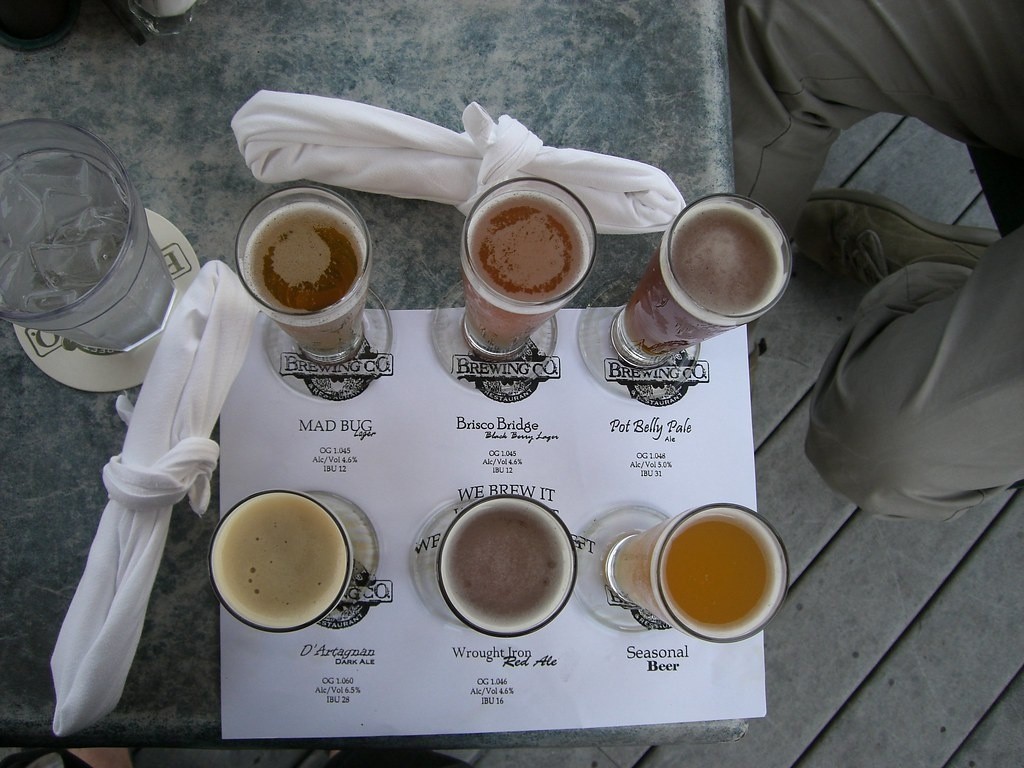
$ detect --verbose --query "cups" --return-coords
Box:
[411,495,577,639]
[0,117,177,352]
[235,185,393,398]
[579,500,790,643]
[429,176,597,392]
[576,195,794,398]
[209,491,382,635]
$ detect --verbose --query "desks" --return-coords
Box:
[0,0,748,748]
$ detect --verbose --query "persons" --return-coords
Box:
[726,0,1024,524]
